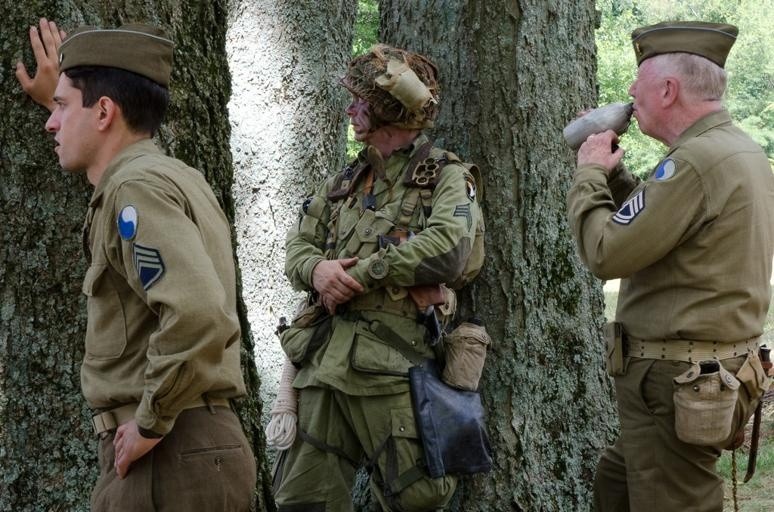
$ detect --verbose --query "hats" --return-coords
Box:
[57,23,174,89]
[629,20,739,68]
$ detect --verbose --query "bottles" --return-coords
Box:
[562,101,634,151]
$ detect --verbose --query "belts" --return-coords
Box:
[621,335,760,360]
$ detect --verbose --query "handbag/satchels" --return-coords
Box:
[440,320,492,394]
[407,366,493,478]
[671,363,741,446]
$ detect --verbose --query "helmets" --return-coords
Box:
[335,44,440,132]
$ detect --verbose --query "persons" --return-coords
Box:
[564,20,774,512]
[272,42,485,512]
[14,16,256,512]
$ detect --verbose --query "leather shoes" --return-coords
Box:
[90,390,234,436]
